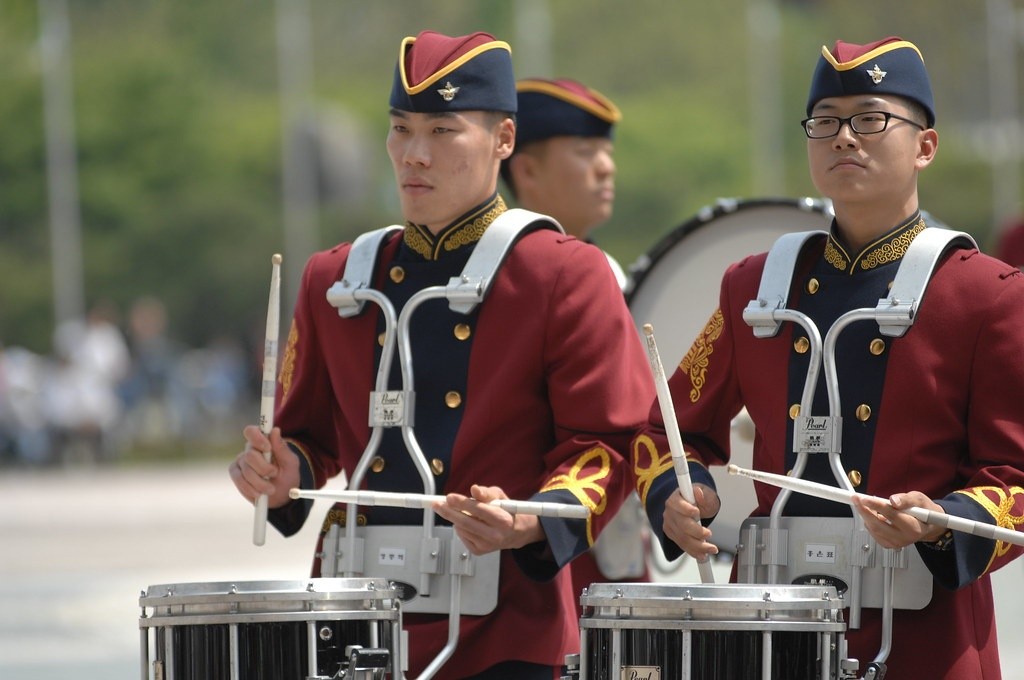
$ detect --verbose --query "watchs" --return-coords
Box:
[923,531,955,552]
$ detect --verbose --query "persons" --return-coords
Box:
[0,293,268,466]
[630,36,1024,680]
[498,79,653,680]
[229,32,658,680]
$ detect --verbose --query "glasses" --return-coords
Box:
[800,111,925,140]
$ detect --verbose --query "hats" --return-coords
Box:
[507,77,623,138]
[390,30,518,114]
[807,37,937,128]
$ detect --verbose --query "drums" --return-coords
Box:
[138,578,403,680]
[576,581,848,680]
[582,187,952,584]
